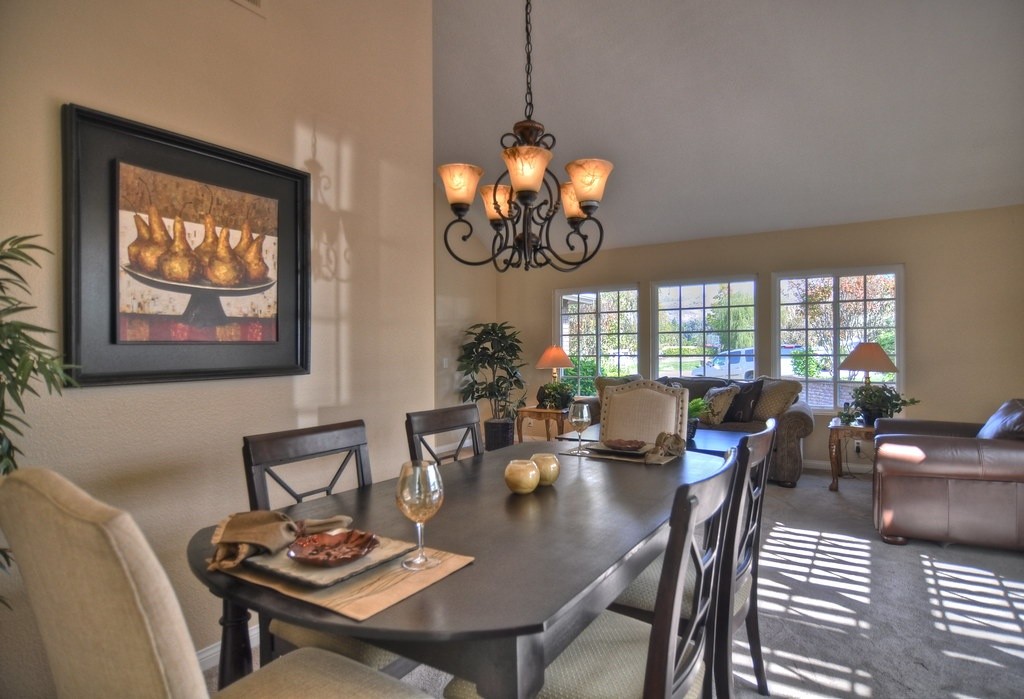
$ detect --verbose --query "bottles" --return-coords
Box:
[504,460,540,494]
[530,453,561,485]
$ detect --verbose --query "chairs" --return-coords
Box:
[598,380,689,442]
[873,399,1024,550]
[0,403,775,699]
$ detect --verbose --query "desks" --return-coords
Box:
[517,404,569,443]
[596,381,814,487]
[828,416,874,490]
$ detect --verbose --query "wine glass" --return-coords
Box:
[395,460,444,572]
[568,403,592,456]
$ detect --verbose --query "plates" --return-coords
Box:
[287,530,380,569]
[603,438,647,451]
[244,528,420,589]
[585,442,655,456]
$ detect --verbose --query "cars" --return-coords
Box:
[691,344,832,381]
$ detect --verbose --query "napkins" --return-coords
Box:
[206,510,354,572]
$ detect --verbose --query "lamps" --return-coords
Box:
[838,343,899,387]
[439,0,614,272]
[537,347,573,382]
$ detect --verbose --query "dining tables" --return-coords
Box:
[186,441,726,699]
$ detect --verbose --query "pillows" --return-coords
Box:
[595,375,801,424]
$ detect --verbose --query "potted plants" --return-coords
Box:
[537,383,574,409]
[456,320,527,450]
[838,385,921,426]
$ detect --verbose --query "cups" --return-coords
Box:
[832,418,840,426]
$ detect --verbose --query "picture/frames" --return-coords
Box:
[61,103,310,384]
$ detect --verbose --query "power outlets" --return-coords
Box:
[854,440,862,452]
